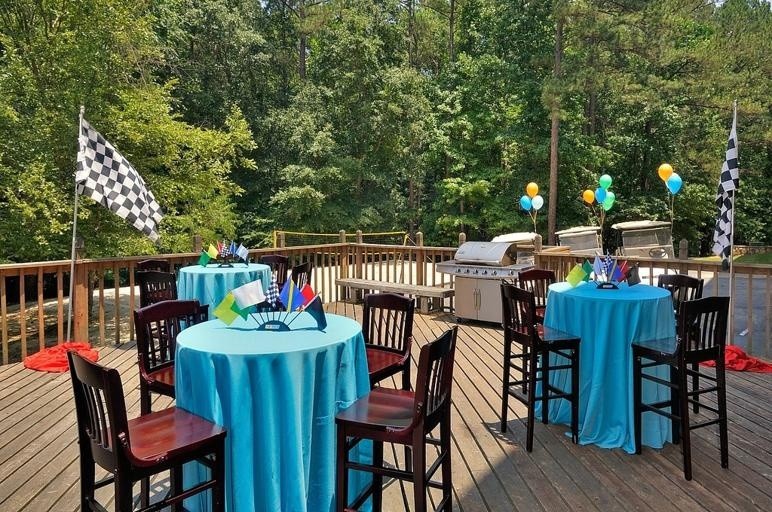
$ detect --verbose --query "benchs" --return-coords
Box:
[335,279,455,315]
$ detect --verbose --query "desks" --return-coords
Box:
[176,312,362,512]
[179,264,271,331]
[548,284,671,454]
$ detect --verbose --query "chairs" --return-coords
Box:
[363,293,415,472]
[134,299,209,416]
[336,325,458,512]
[658,275,704,414]
[500,284,581,453]
[258,301,286,312]
[632,296,730,481]
[518,269,556,395]
[138,271,181,357]
[292,262,312,291]
[137,259,170,307]
[260,255,289,293]
[67,350,227,512]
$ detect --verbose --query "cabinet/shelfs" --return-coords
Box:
[455,276,502,323]
